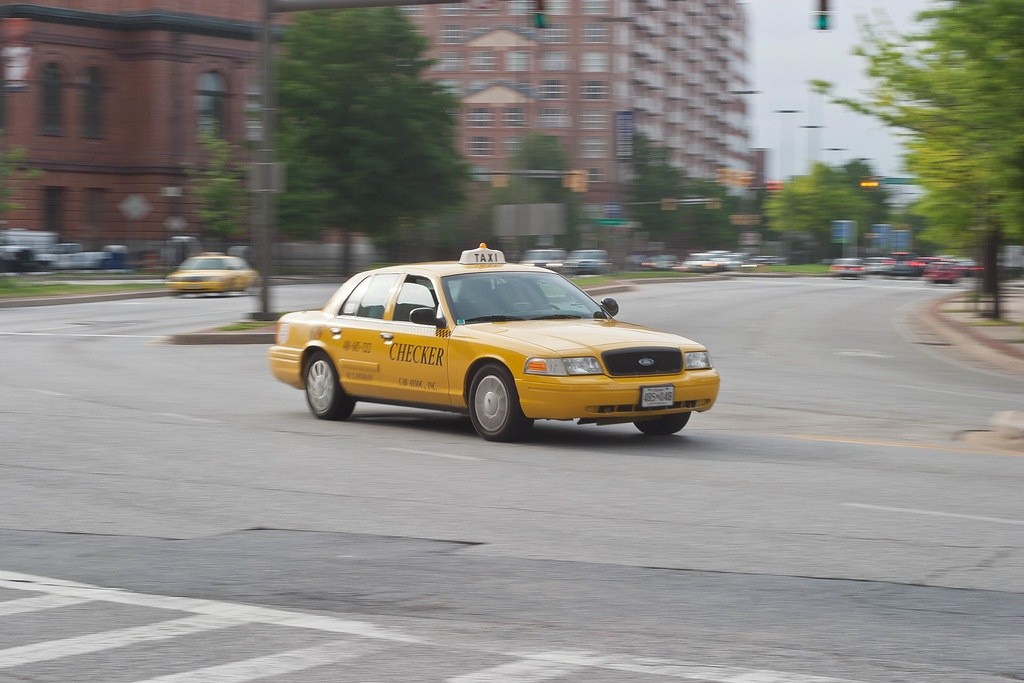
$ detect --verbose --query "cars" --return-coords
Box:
[518,246,984,287]
[164,250,262,293]
[266,238,720,443]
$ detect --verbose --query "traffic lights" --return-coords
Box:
[532,0,551,28]
[817,0,830,32]
